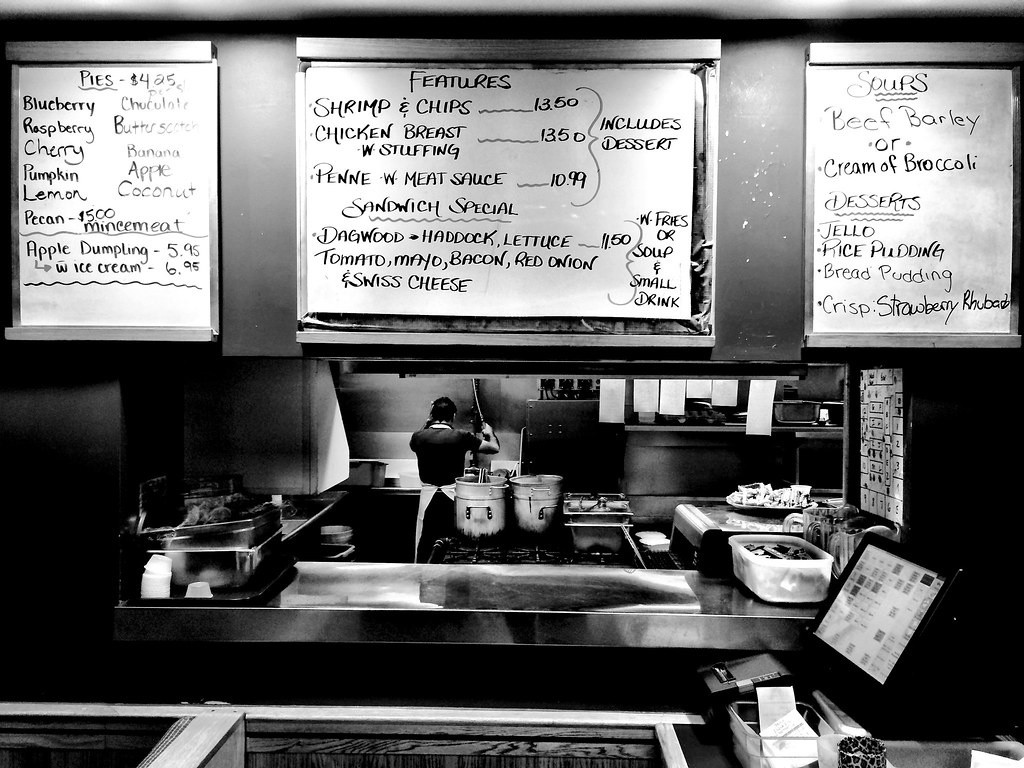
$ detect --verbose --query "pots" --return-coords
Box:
[455,475,509,544]
[507,474,563,545]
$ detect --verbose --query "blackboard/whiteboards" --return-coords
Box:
[293,36,722,347]
[804,42,1023,349]
[1,40,221,342]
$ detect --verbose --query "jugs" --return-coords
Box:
[782,508,900,581]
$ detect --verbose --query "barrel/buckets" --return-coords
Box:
[321,525,353,545]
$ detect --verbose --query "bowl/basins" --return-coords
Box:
[140,554,173,598]
[773,401,821,423]
[184,581,213,598]
[817,734,886,768]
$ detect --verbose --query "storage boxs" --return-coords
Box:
[729,534,835,603]
[823,401,843,424]
[729,701,836,768]
[340,461,389,486]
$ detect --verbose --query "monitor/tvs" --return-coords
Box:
[802,531,968,707]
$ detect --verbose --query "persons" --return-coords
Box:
[409,397,500,563]
